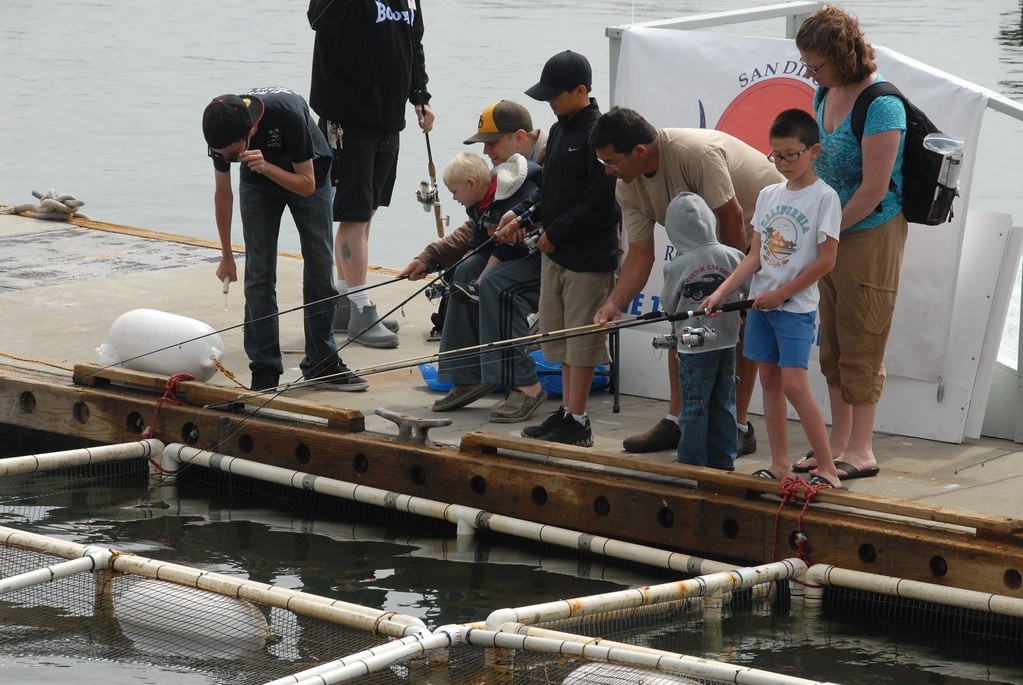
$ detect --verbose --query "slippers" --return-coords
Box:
[748,469,775,481]
[808,476,849,491]
[792,450,820,473]
[812,460,880,481]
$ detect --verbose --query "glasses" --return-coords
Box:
[208,136,249,163]
[799,56,835,74]
[595,153,634,170]
[767,144,815,164]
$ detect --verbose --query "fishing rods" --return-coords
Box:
[144,199,546,495]
[411,43,451,239]
[81,262,457,377]
[202,297,791,410]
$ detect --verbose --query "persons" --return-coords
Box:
[394,100,549,423]
[587,105,788,458]
[443,151,543,304]
[699,108,849,492]
[305,0,436,345]
[660,191,753,473]
[495,49,622,448]
[792,6,910,480]
[201,86,370,396]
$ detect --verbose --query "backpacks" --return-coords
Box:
[816,79,964,225]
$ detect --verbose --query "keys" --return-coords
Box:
[337,128,344,149]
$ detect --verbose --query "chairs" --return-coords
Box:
[497,276,622,414]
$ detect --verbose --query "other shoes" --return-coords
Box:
[625,418,683,451]
[433,382,500,409]
[738,421,759,458]
[491,388,548,422]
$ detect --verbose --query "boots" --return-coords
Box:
[347,299,399,347]
[333,296,399,336]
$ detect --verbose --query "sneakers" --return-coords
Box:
[520,405,567,438]
[251,374,279,394]
[538,413,596,446]
[304,362,370,390]
[450,280,481,307]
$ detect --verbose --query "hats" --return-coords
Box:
[523,49,592,101]
[463,99,532,145]
[203,94,265,150]
